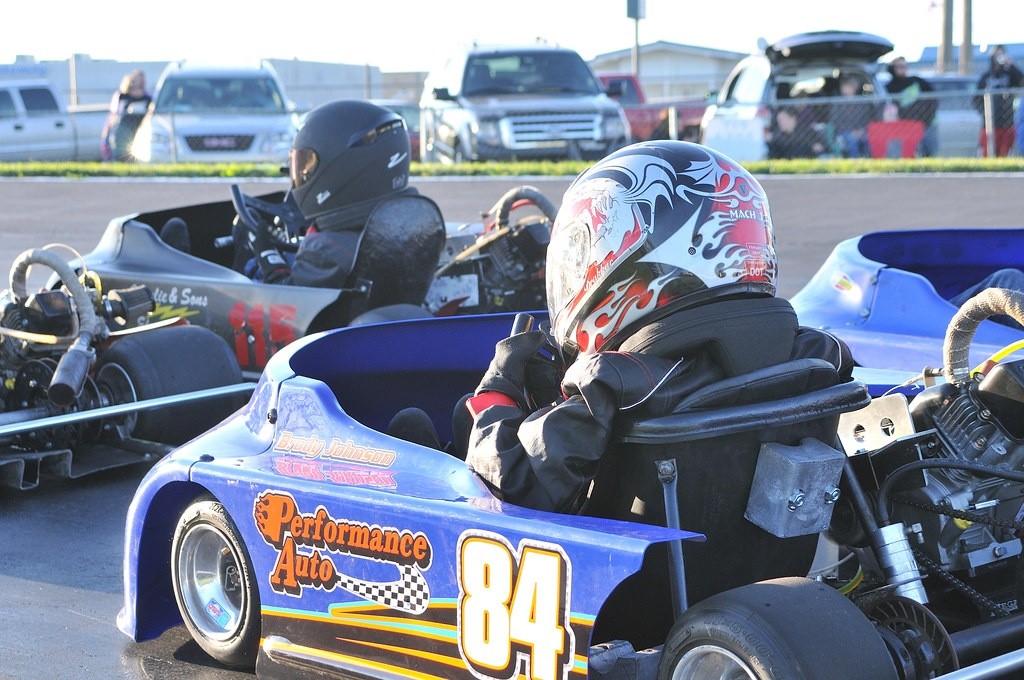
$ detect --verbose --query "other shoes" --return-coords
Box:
[159,217,192,254]
[387,407,440,452]
[451,393,474,461]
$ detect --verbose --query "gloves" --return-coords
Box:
[472,328,567,414]
[247,220,290,277]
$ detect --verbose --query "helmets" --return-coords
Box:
[544,138,781,360]
[291,100,410,219]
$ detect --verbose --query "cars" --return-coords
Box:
[875,71,1023,158]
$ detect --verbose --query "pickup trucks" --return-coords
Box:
[0,80,111,164]
[596,74,708,144]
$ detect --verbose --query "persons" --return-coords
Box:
[971,46,1024,157]
[159,98,418,288]
[386,140,854,517]
[763,57,939,158]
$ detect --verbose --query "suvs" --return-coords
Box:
[698,30,894,158]
[418,36,633,164]
[130,60,298,164]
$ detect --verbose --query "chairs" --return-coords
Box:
[331,196,447,324]
[585,358,872,653]
